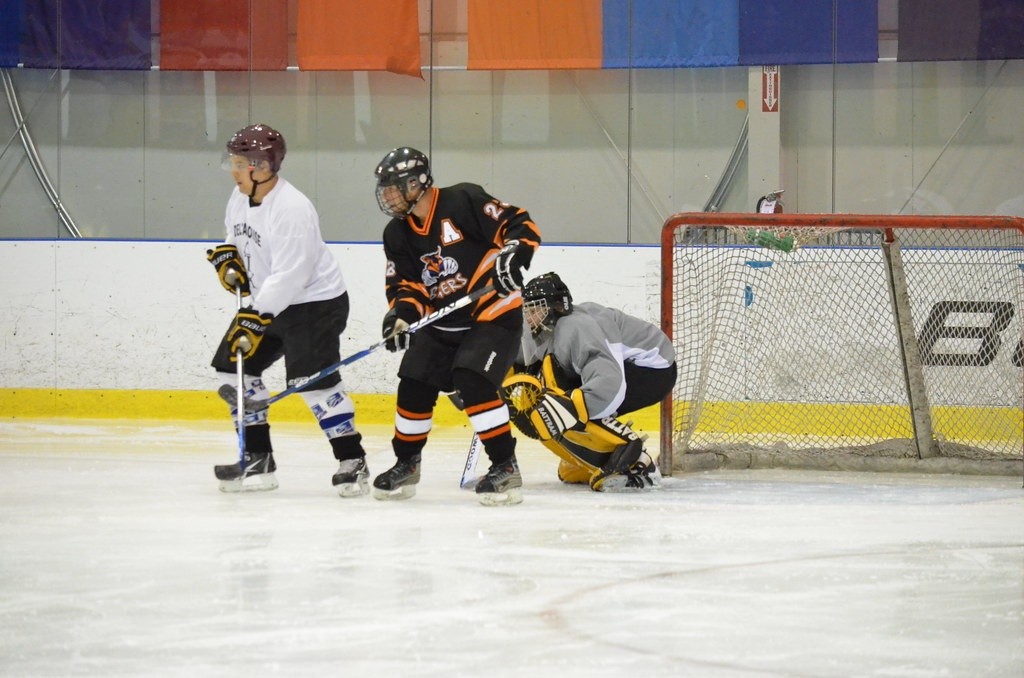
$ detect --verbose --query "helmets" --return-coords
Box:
[521,272,574,339]
[375,147,433,219]
[221,124,286,173]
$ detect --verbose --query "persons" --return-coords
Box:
[205,123,370,486]
[372,145,542,494]
[495,269,677,492]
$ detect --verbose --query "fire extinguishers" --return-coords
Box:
[755,189,786,214]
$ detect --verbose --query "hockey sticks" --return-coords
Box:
[216,286,493,413]
[213,283,246,480]
[460,432,479,486]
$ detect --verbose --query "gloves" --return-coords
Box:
[207,244,251,297]
[382,301,421,352]
[492,239,534,298]
[226,308,274,363]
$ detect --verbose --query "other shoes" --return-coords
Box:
[590,447,663,492]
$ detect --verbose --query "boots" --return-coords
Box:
[371,437,427,500]
[215,423,279,493]
[476,437,524,506]
[330,432,370,497]
